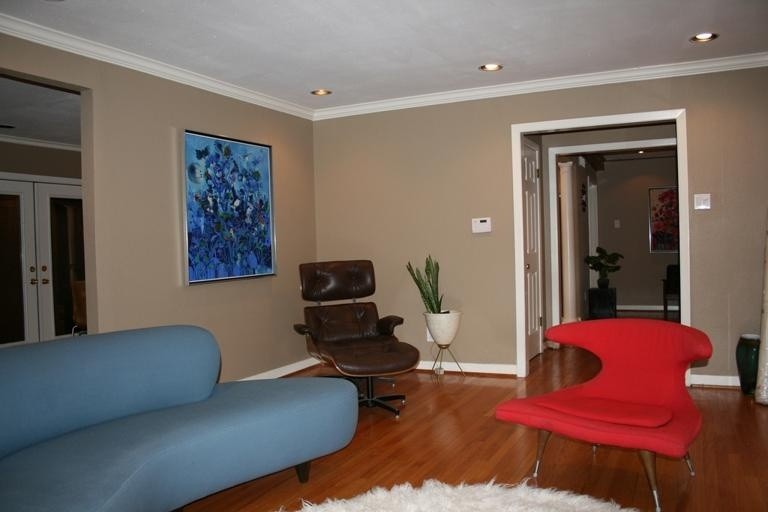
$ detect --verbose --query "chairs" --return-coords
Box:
[492,316,713,512]
[293,259,421,418]
[661,264,680,320]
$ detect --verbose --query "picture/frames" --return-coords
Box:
[184,128,276,284]
[647,186,678,254]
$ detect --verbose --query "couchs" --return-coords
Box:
[0,322,361,512]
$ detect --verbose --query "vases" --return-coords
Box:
[734,333,761,395]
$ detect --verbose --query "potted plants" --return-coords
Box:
[585,246,626,286]
[406,251,461,349]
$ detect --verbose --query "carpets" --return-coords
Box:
[281,476,637,512]
